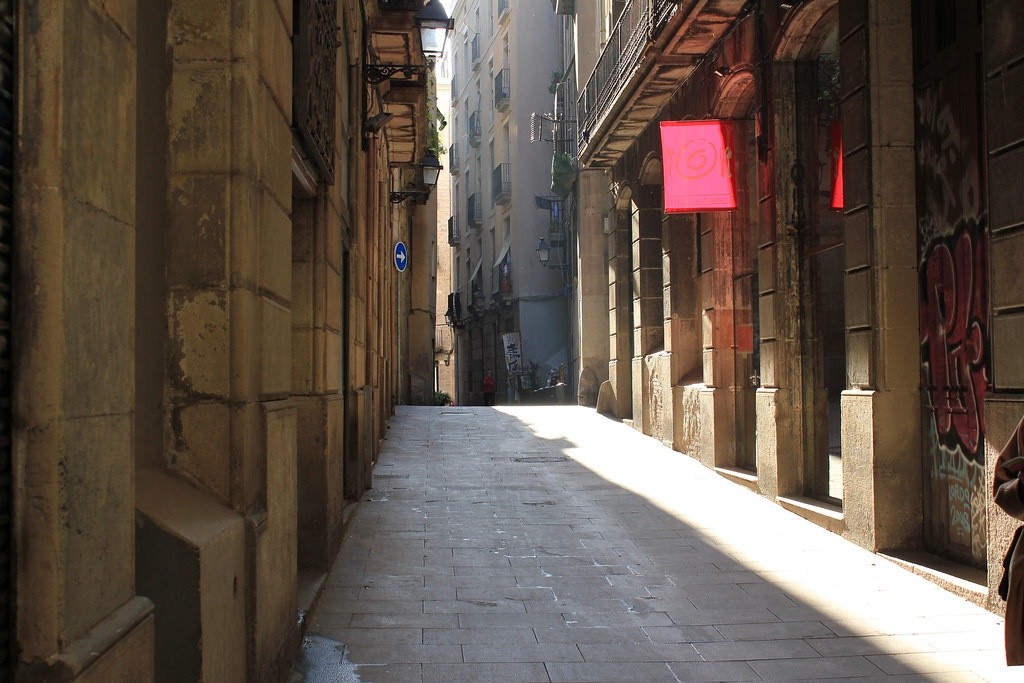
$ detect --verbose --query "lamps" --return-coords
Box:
[714,65,733,76]
[390,150,443,213]
[536,236,571,270]
[473,288,496,310]
[365,0,455,86]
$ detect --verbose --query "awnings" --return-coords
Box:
[468,257,482,284]
[492,238,510,268]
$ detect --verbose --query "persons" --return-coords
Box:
[992,417,1024,667]
[483,371,496,405]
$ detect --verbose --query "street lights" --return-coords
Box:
[474,287,488,392]
